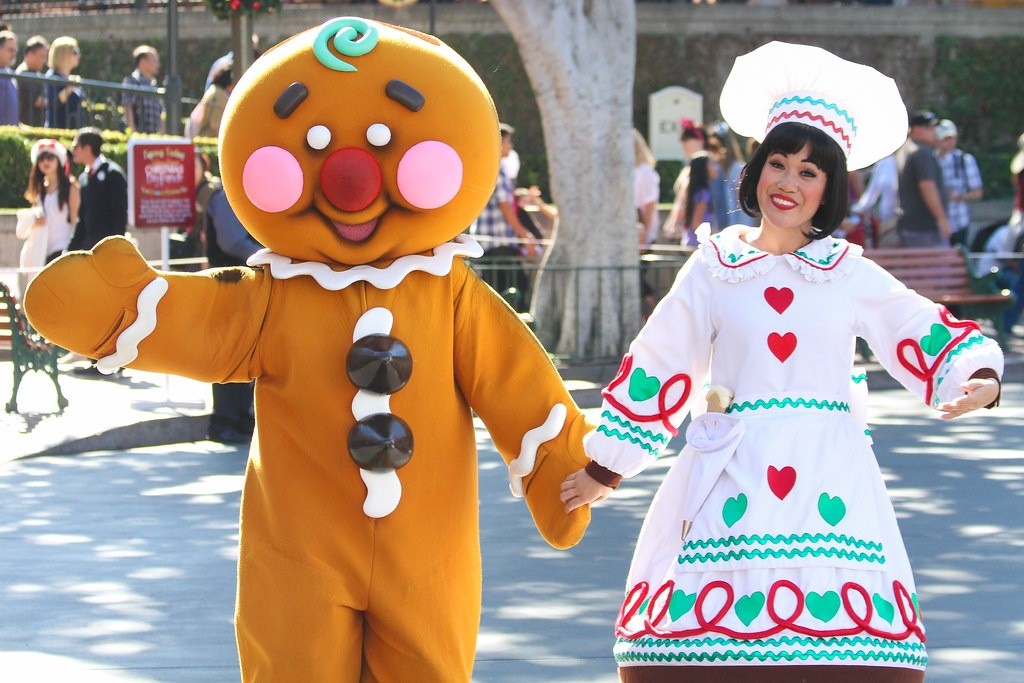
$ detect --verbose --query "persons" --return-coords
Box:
[198,42,263,137]
[205,188,264,443]
[847,112,984,248]
[561,41,1004,683]
[16,132,127,375]
[121,45,164,135]
[168,151,220,273]
[631,120,756,313]
[974,132,1024,339]
[473,125,555,312]
[0,21,85,127]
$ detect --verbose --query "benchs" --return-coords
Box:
[0,282,70,413]
[857,247,1018,357]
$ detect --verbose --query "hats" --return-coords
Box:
[934,119,958,142]
[1009,132,1024,175]
[30,138,69,168]
[719,39,910,172]
[909,111,940,128]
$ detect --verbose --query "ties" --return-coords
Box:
[87,168,93,180]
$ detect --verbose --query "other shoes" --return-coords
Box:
[74,368,114,379]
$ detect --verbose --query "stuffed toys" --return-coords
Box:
[23,15,598,683]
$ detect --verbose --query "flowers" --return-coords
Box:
[203,0,282,22]
